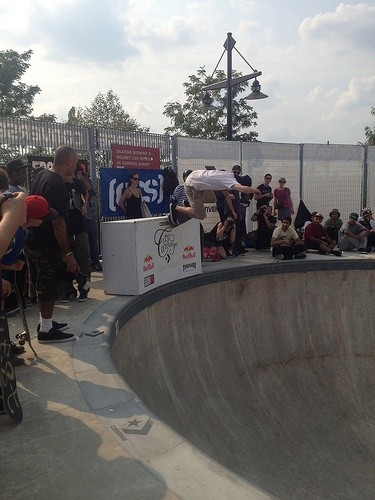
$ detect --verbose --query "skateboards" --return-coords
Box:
[166,212,192,228]
[0,295,24,424]
[14,270,38,359]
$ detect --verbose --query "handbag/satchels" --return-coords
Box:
[275,200,283,209]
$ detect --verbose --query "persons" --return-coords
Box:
[0,159,102,366]
[23,146,79,342]
[301,206,375,257]
[120,172,144,219]
[271,216,307,259]
[0,192,27,414]
[273,177,294,226]
[254,174,273,210]
[205,165,253,259]
[169,170,262,223]
[172,170,193,207]
[247,205,277,249]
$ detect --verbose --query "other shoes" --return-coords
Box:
[358,248,368,254]
[275,254,284,259]
[10,345,26,365]
[332,250,344,257]
[295,253,306,259]
[60,290,78,303]
[78,293,88,302]
[169,203,178,224]
[182,200,192,218]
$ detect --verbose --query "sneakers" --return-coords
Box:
[38,328,76,344]
[37,320,68,334]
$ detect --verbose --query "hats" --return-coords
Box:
[349,212,359,221]
[6,159,31,172]
[232,165,242,170]
[362,207,373,217]
[26,195,59,220]
[234,174,252,187]
[329,209,340,218]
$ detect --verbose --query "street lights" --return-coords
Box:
[200,32,269,142]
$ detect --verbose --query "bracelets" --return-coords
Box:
[65,252,72,257]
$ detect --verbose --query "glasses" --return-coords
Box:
[234,171,240,174]
[133,177,141,181]
[265,177,271,180]
[279,181,285,183]
